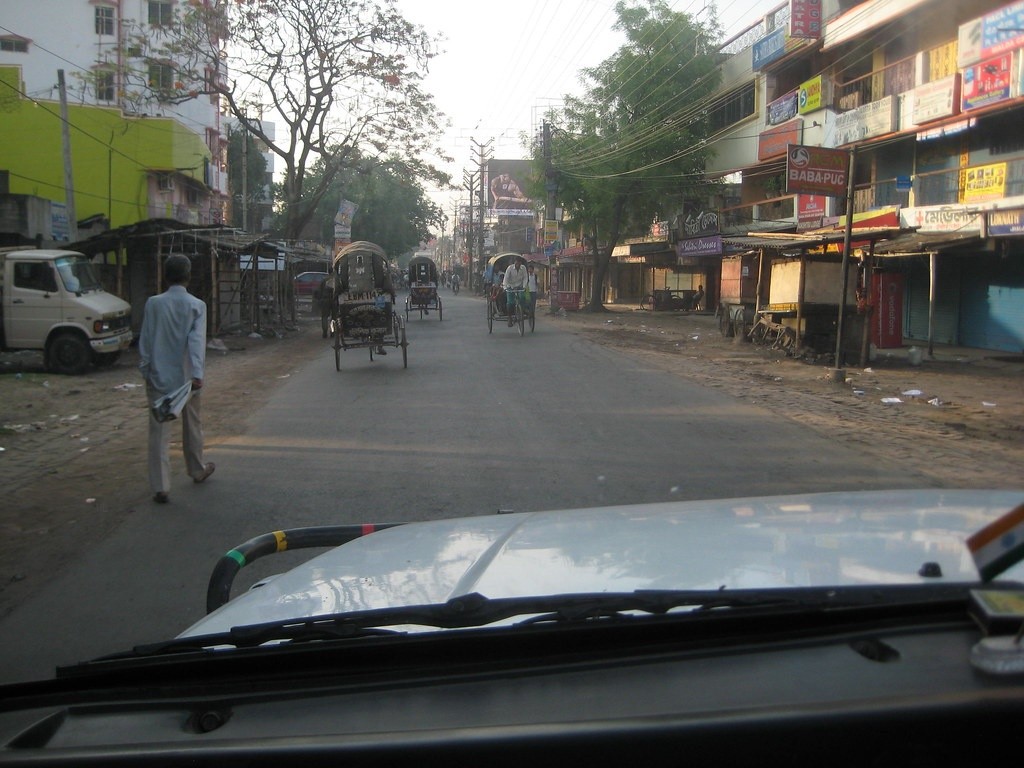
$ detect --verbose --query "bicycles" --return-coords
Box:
[443,277,460,297]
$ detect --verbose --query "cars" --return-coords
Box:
[293,271,328,293]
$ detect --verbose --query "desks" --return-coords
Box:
[747,317,801,357]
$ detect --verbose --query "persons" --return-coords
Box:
[692,285,702,299]
[139,254,216,503]
[492,262,507,316]
[316,265,343,338]
[437,269,453,288]
[451,272,461,292]
[490,167,529,210]
[526,265,540,308]
[490,274,504,300]
[392,266,409,289]
[502,257,529,327]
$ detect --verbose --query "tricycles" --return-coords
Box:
[403,249,443,322]
[328,240,410,372]
[484,249,536,337]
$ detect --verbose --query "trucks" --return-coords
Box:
[0,244,134,376]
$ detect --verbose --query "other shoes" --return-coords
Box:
[153,490,168,503]
[194,461,215,483]
[507,320,513,327]
[378,348,387,355]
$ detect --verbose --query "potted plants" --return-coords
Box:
[779,174,787,196]
[765,175,780,199]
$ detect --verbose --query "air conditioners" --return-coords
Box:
[668,231,677,243]
[161,174,176,192]
